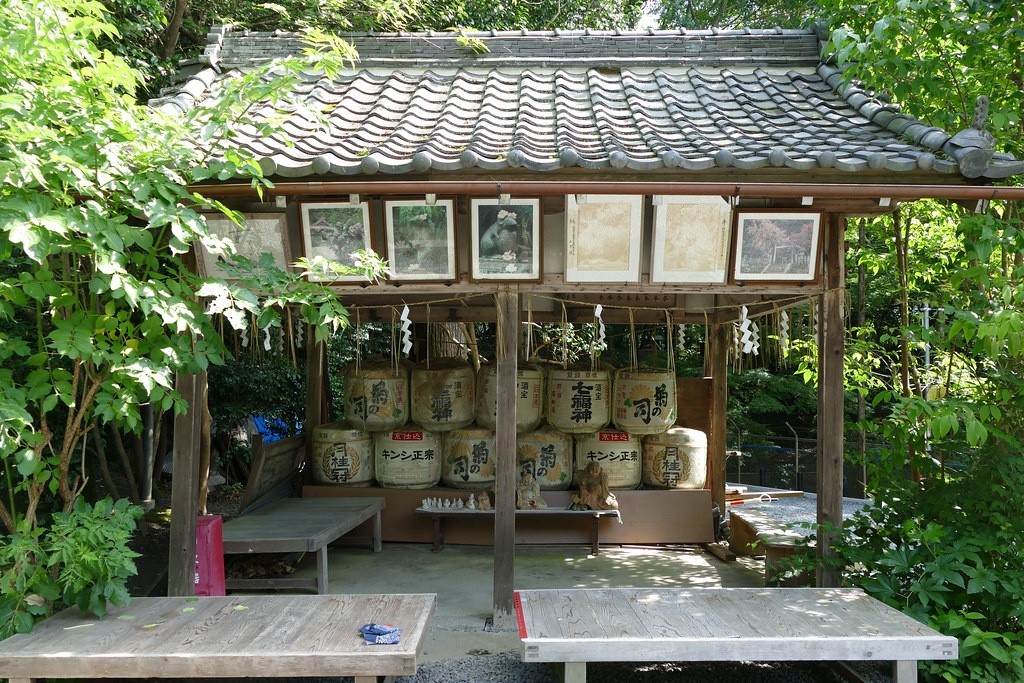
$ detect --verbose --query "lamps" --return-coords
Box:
[729,205,826,286]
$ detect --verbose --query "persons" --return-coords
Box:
[577,461,619,510]
[519,475,547,511]
[477,491,491,509]
[422,494,475,509]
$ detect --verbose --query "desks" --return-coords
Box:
[0,593,438,683]
[513,588,959,683]
[220,494,388,595]
[414,505,623,556]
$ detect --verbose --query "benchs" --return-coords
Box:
[728,508,816,588]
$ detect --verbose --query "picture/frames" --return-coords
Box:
[380,194,462,286]
[563,193,646,286]
[172,201,305,288]
[295,196,379,286]
[648,194,732,286]
[466,193,546,286]
